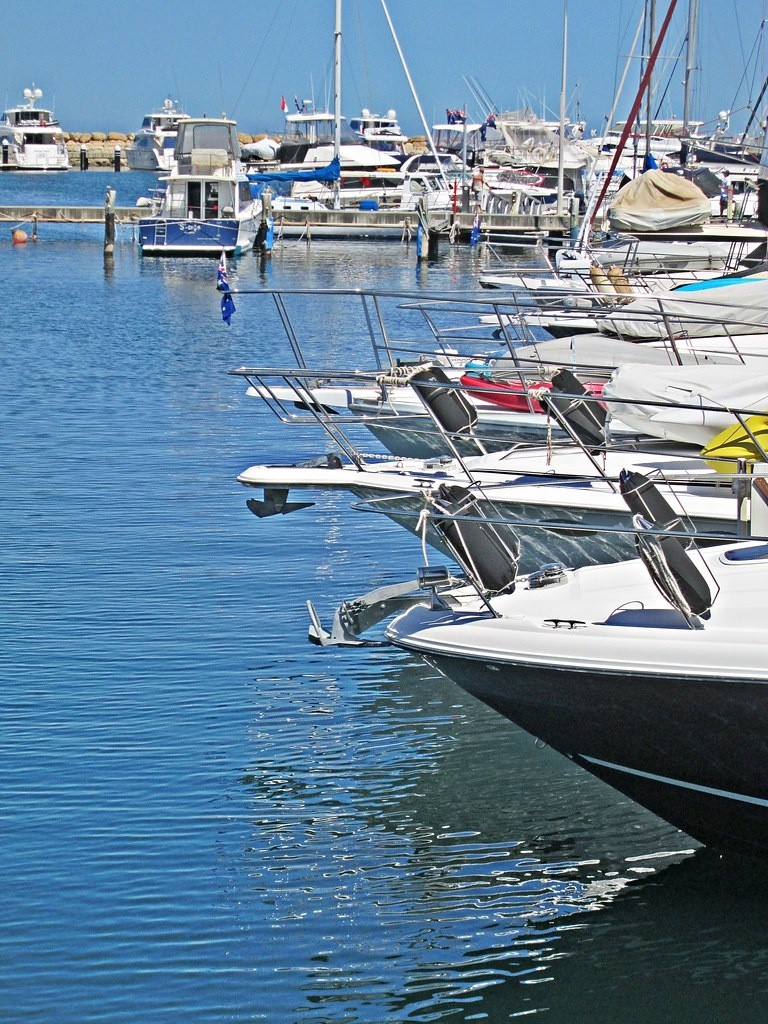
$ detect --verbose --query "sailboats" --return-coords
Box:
[126,0,768,866]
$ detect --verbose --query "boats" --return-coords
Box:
[0,82,73,170]
[123,94,195,171]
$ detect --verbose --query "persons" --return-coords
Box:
[718,170,731,216]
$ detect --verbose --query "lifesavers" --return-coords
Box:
[376,168,396,172]
[660,161,669,170]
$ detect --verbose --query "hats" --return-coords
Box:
[722,170,730,174]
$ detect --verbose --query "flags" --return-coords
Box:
[470,212,480,247]
[281,96,308,113]
[217,251,236,326]
[445,108,497,142]
